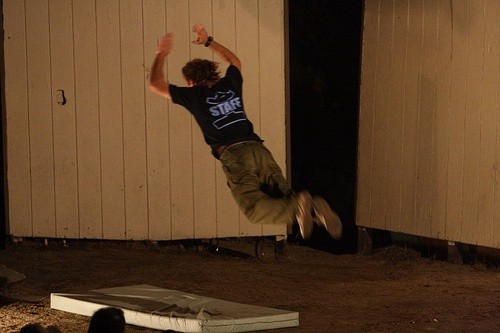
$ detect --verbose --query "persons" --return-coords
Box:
[148,24,343,239]
[19,308,126,333]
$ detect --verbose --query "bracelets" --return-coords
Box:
[205,36,214,47]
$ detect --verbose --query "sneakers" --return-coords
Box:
[311,195,342,240]
[296,190,312,240]
